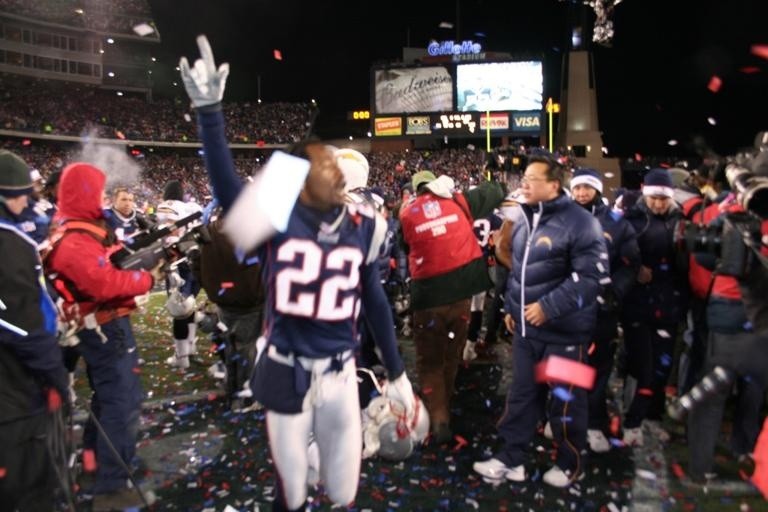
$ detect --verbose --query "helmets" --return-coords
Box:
[357,367,430,460]
[334,148,370,194]
[165,286,196,320]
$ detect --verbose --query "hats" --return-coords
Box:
[569,168,603,193]
[411,170,436,194]
[163,179,183,201]
[641,168,674,198]
[0,150,34,195]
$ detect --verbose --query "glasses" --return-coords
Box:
[521,175,547,182]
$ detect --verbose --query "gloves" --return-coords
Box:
[168,272,185,289]
[387,371,414,416]
[179,34,229,109]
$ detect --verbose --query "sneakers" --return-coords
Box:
[622,426,670,447]
[541,465,585,488]
[472,456,526,482]
[585,429,609,453]
[668,459,715,491]
[93,485,155,512]
[166,352,190,369]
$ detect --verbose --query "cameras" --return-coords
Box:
[685,208,762,259]
[110,211,212,272]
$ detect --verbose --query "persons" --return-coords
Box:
[102,187,158,241]
[157,179,203,368]
[462,215,501,362]
[168,36,421,511]
[393,170,509,445]
[48,162,170,512]
[564,168,641,455]
[472,152,610,487]
[25,164,48,238]
[0,149,76,512]
[686,165,768,482]
[619,169,694,446]
[332,148,382,214]
